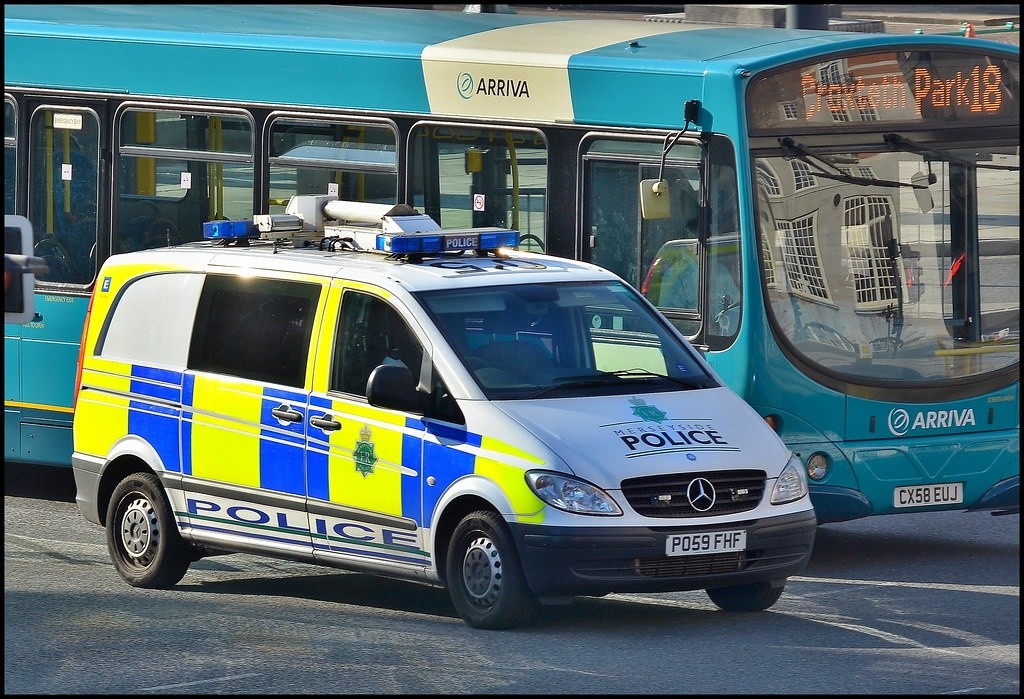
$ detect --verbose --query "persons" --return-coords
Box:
[345,303,422,397]
[657,216,741,336]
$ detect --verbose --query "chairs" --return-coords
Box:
[473,340,549,383]
[34,198,186,284]
[343,301,395,391]
